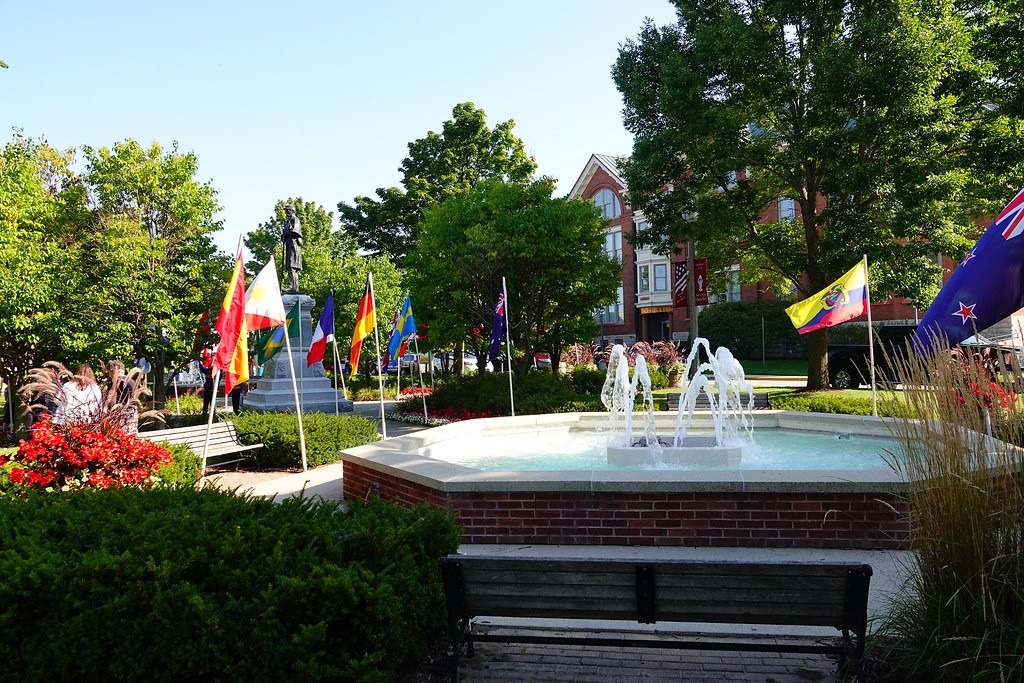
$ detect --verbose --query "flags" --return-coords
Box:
[909,188,1024,371]
[784,258,869,336]
[135,248,418,397]
[488,283,508,368]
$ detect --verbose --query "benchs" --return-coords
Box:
[138,421,265,472]
[666,393,772,410]
[438,554,873,683]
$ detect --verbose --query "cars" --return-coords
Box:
[343,354,569,376]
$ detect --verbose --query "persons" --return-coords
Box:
[198,359,224,416]
[49,363,103,440]
[281,204,304,294]
[231,383,255,417]
[104,359,138,460]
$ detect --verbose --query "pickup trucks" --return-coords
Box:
[827,323,1024,390]
